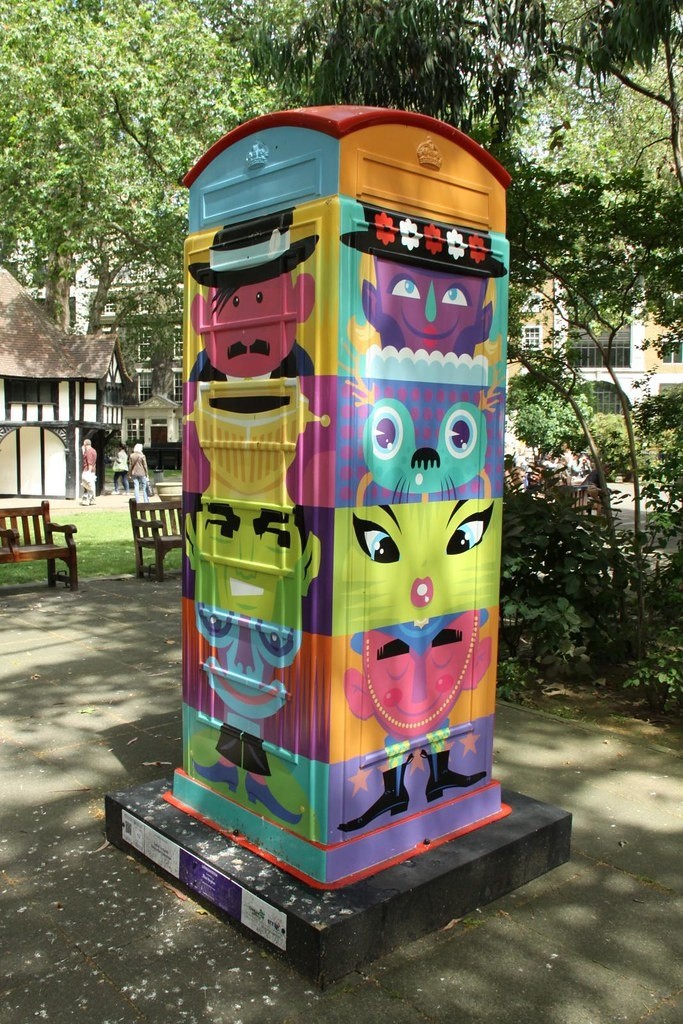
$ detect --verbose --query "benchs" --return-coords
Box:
[129,498,181,582]
[0,500,79,592]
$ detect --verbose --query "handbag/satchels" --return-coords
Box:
[81,471,97,491]
[147,481,154,497]
[112,460,125,472]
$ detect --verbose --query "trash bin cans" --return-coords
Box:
[153,470,163,487]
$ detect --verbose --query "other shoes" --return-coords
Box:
[111,491,119,495]
[122,492,129,496]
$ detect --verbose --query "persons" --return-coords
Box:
[129,442,151,503]
[504,443,592,503]
[112,442,130,495]
[82,440,97,505]
